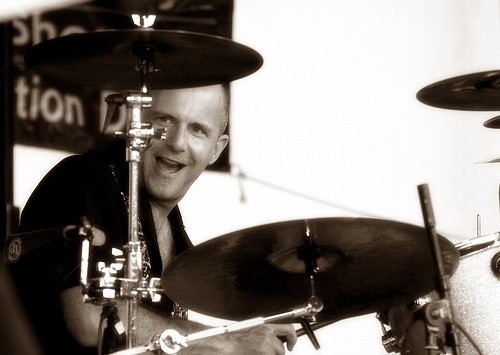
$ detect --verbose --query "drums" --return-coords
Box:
[416,230,500,355]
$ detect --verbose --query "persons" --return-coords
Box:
[17,83,297,355]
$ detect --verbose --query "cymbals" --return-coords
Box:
[417,70,500,112]
[26,29,263,91]
[483,115,500,129]
[161,216,460,324]
